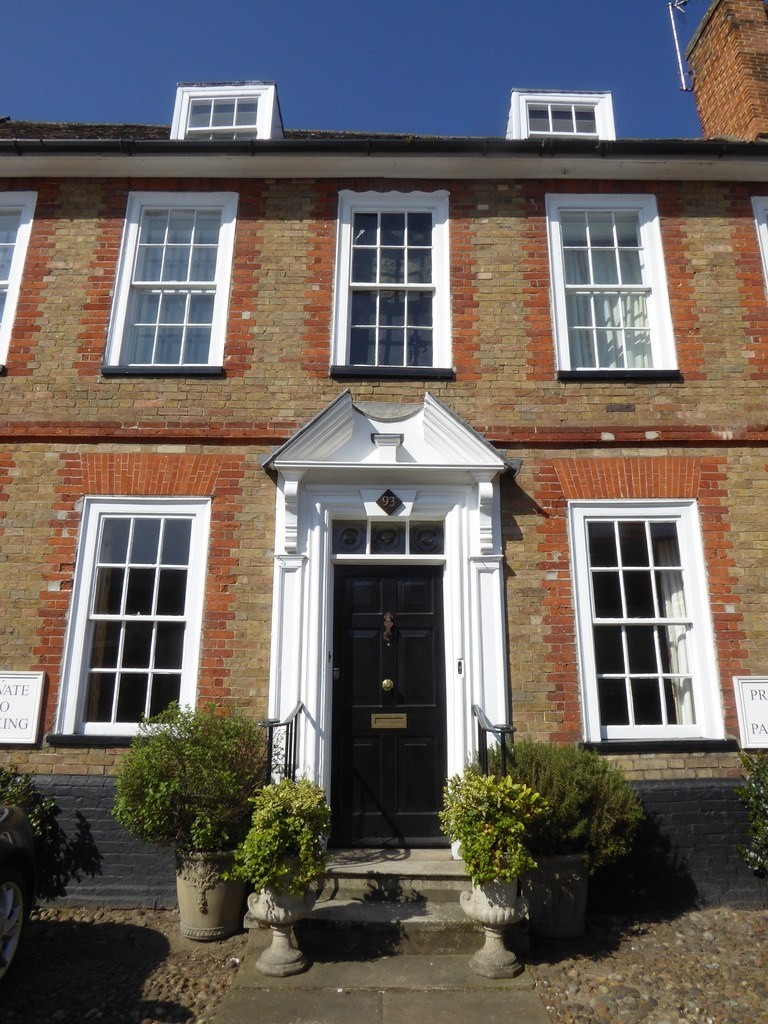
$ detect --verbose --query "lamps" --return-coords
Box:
[371,433,405,448]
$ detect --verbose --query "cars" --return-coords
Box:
[0,802,36,990]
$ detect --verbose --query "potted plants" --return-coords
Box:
[112,700,331,976]
[437,733,645,978]
